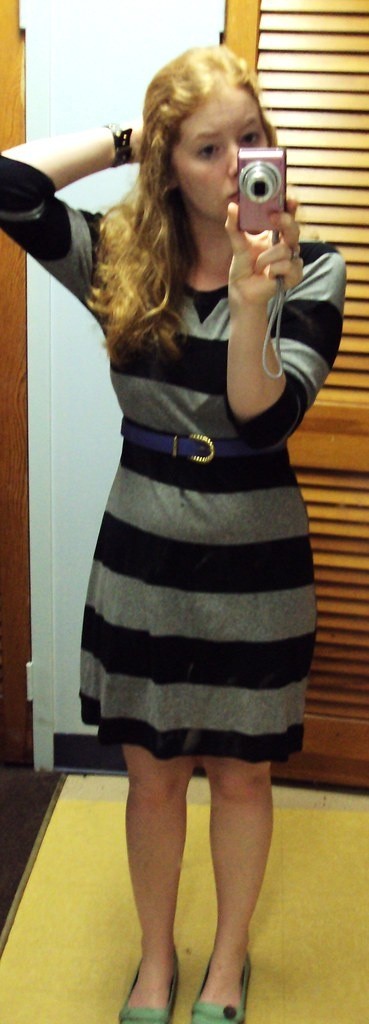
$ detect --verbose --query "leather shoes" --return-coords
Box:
[119,950,178,1024]
[191,948,251,1024]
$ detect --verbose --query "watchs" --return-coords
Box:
[103,122,134,168]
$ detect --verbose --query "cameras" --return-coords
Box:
[237,146,286,230]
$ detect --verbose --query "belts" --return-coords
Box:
[120,417,288,462]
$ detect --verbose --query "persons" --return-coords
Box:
[1,45,346,1024]
